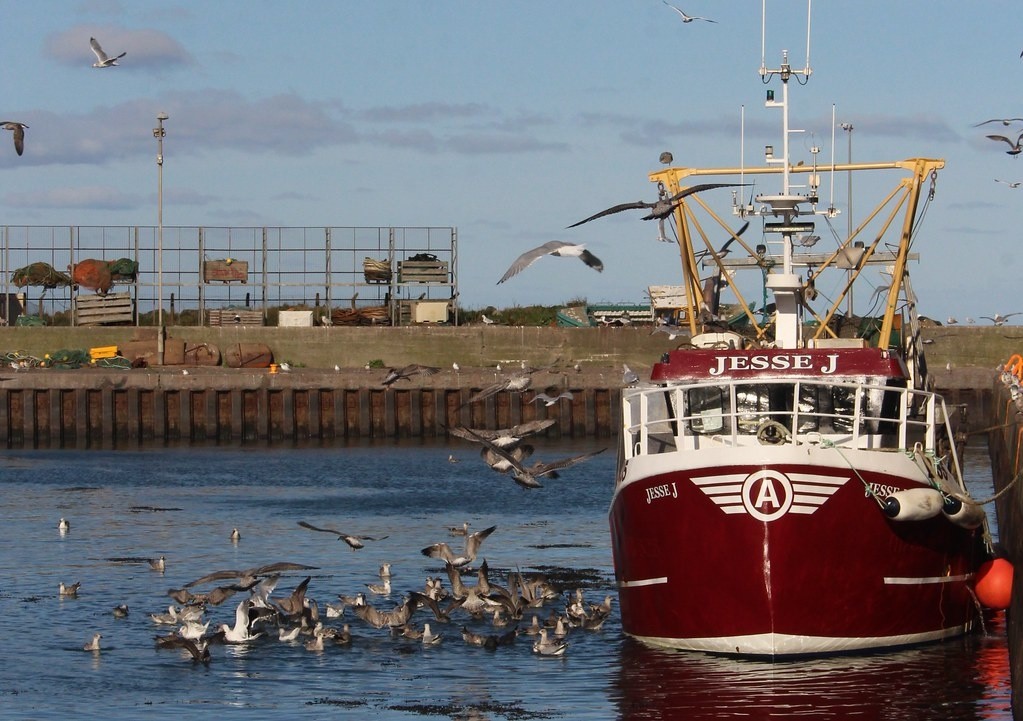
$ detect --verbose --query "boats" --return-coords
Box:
[606,110,1014,663]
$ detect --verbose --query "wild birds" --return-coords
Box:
[365,362,371,371]
[56,556,612,664]
[322,316,334,327]
[497,242,603,284]
[296,521,388,553]
[382,364,439,390]
[0,121,29,157]
[89,38,127,68]
[973,118,1022,188]
[451,314,690,408]
[11,361,33,372]
[335,364,341,372]
[947,312,1023,328]
[662,1,720,24]
[422,521,496,567]
[567,183,753,228]
[280,363,291,373]
[59,518,69,528]
[231,528,241,539]
[448,421,609,489]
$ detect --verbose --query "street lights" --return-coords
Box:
[152,110,170,362]
